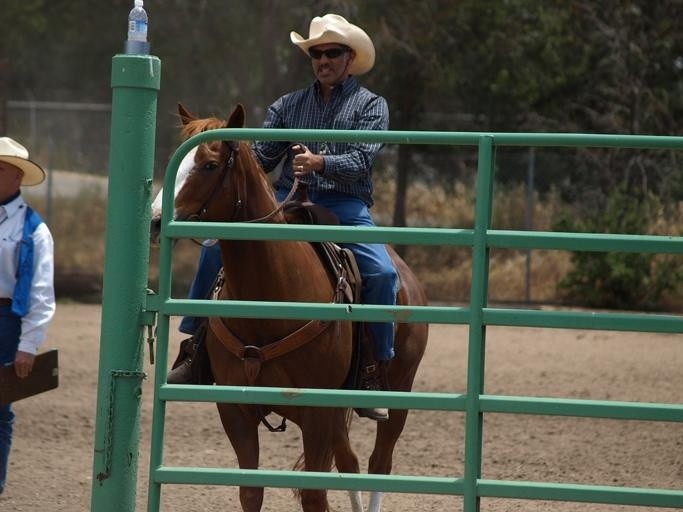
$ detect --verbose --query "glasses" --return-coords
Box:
[307,46,352,60]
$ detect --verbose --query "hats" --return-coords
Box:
[290,13,376,76]
[0,137,47,187]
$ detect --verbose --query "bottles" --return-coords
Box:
[126,0,150,43]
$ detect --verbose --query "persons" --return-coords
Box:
[166,13,401,423]
[0,135,57,493]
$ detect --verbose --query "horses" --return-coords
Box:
[149,101,430,512]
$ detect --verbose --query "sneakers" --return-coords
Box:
[353,360,389,420]
[166,340,199,383]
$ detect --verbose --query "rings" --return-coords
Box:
[298,165,304,172]
